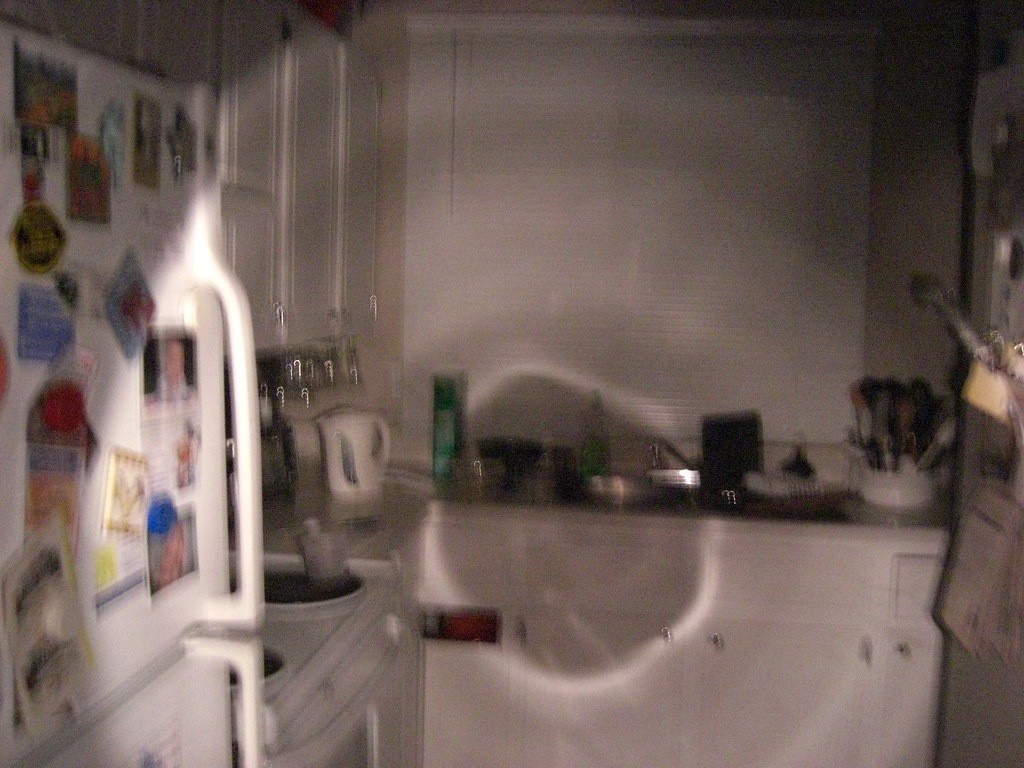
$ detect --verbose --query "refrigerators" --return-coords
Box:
[0,17,268,768]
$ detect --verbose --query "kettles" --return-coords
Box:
[317,414,389,503]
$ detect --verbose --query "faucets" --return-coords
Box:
[640,428,702,497]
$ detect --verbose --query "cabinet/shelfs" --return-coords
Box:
[0,0,350,348]
[420,517,945,768]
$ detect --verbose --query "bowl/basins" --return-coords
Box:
[862,468,931,504]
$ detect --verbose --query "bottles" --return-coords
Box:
[583,394,610,474]
